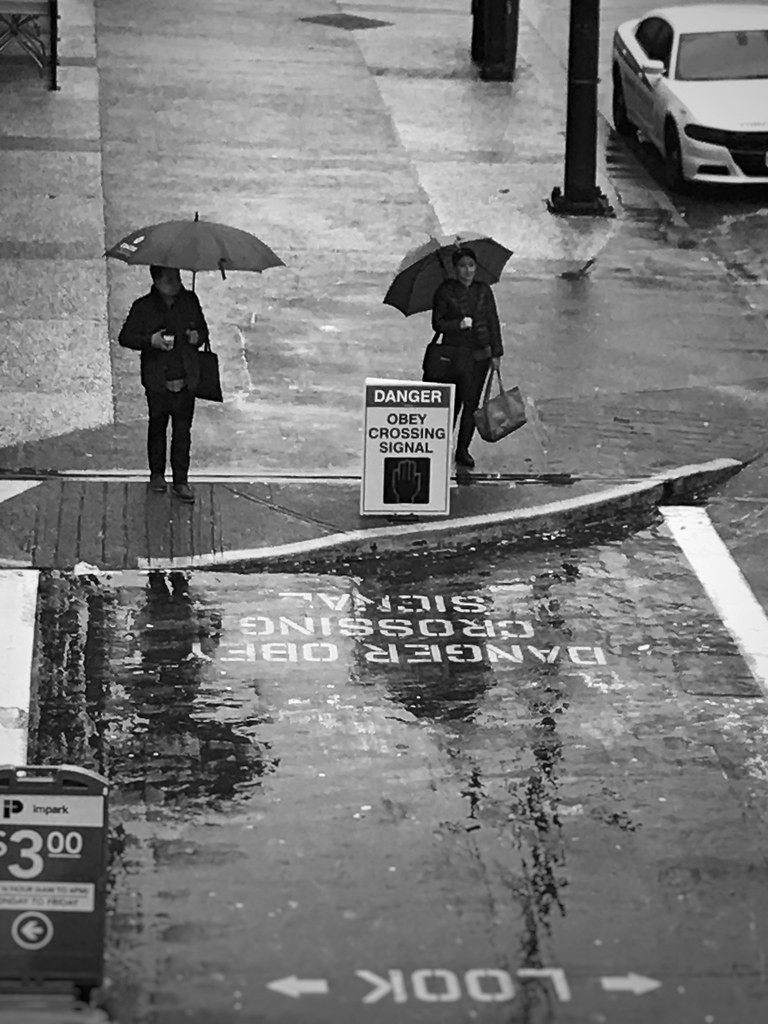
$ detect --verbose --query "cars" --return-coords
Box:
[613,5,768,192]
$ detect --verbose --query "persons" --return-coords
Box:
[432,247,504,468]
[116,264,209,503]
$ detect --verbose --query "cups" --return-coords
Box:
[162,331,174,349]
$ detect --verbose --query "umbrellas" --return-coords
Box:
[99,210,288,330]
[382,230,514,317]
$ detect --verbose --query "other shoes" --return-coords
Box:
[455,449,476,468]
[150,472,168,492]
[171,483,195,503]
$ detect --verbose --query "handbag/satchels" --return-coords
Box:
[473,367,529,443]
[422,323,466,381]
[191,321,223,403]
